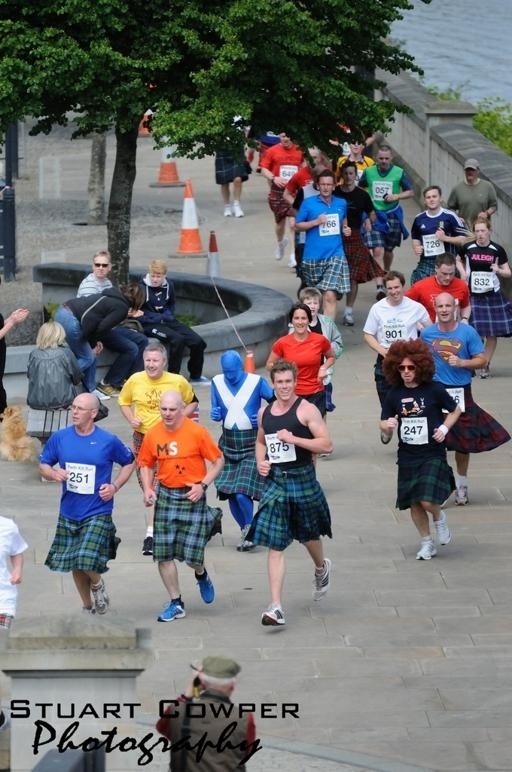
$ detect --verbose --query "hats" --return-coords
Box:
[189,655,244,696]
[464,158,480,171]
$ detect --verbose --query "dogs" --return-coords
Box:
[0,405,42,463]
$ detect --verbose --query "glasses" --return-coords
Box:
[397,364,418,372]
[93,262,108,267]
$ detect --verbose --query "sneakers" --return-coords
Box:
[91,389,111,401]
[380,432,392,444]
[142,536,154,556]
[480,367,491,380]
[223,203,233,216]
[456,483,470,507]
[194,570,215,605]
[434,511,452,547]
[187,375,212,385]
[157,600,187,623]
[342,311,354,327]
[82,602,96,615]
[260,604,288,628]
[275,237,290,260]
[236,523,256,552]
[312,558,331,599]
[417,537,438,562]
[96,379,121,397]
[89,579,110,616]
[374,287,387,300]
[287,253,297,270]
[233,203,244,217]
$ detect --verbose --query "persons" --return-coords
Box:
[1,117,511,638]
[156,657,257,771]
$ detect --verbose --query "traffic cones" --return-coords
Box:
[135,97,155,136]
[338,120,356,141]
[171,181,209,259]
[243,350,257,376]
[203,232,224,278]
[152,128,183,188]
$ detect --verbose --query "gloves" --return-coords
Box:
[250,414,262,427]
[210,406,222,422]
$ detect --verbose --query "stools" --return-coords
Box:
[40,406,71,444]
